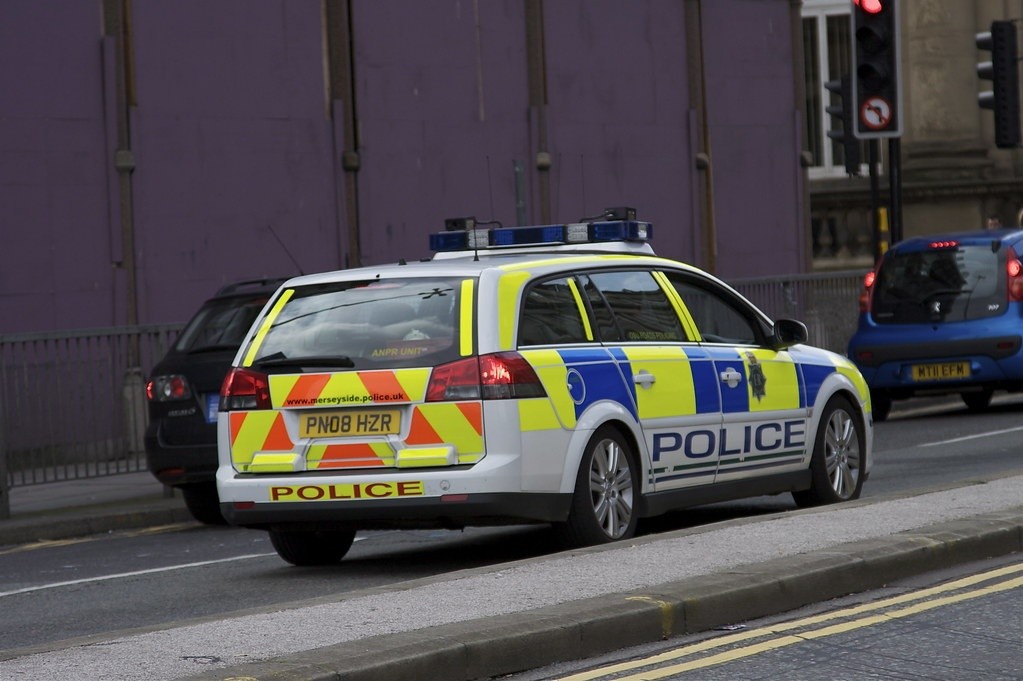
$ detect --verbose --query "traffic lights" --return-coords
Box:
[824,81,861,178]
[975,21,1021,148]
[849,0,903,138]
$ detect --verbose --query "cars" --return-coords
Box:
[847,229,1023,422]
[216,205,875,566]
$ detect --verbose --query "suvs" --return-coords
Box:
[144,275,300,526]
